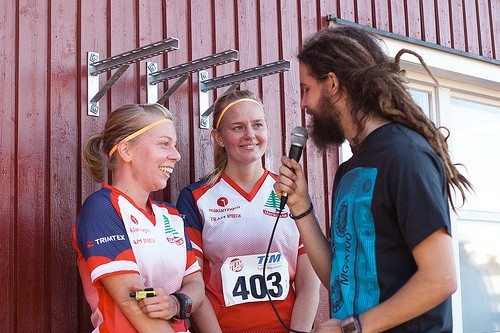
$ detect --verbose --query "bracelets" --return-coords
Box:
[288,203,314,220]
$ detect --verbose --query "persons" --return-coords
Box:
[71,102,207,333]
[176,88,323,333]
[292,25,476,333]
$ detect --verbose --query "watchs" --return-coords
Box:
[340,314,363,333]
[171,292,193,320]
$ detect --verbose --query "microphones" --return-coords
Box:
[280,126,308,209]
